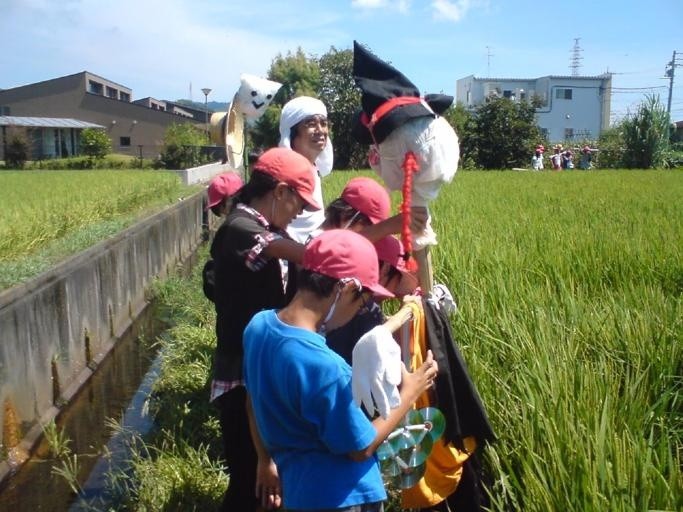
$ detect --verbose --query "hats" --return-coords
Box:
[205,173,242,209]
[255,148,321,211]
[299,177,408,299]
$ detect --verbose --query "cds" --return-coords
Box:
[395,430,433,467]
[391,455,425,490]
[376,425,401,462]
[408,407,446,443]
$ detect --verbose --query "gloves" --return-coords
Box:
[352,327,402,421]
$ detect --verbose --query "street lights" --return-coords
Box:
[202,88,213,139]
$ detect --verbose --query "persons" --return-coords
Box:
[530,142,597,171]
[202,97,439,511]
